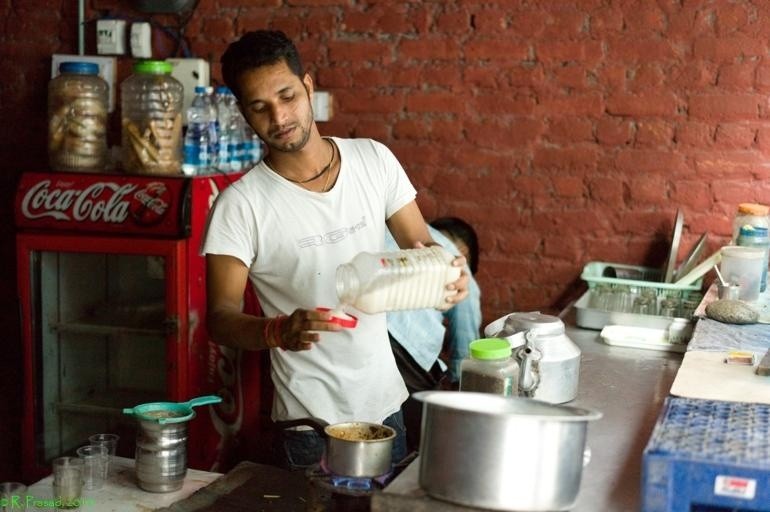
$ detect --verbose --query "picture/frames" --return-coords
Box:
[27,455,225,512]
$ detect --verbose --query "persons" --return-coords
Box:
[369,216,483,448]
[201,31,470,481]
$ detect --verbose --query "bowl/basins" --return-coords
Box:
[265,139,336,195]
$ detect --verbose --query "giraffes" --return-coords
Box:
[255,460,388,512]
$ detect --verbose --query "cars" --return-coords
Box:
[0,404,189,511]
[719,283,738,301]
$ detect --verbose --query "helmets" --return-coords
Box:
[663,208,725,286]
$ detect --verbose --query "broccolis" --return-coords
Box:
[11,169,270,494]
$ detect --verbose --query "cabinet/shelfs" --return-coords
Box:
[591,284,699,327]
[720,202,770,303]
[333,242,464,328]
[461,335,520,401]
[48,61,264,176]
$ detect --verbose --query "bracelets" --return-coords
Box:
[409,387,601,510]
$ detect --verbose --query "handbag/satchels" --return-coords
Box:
[263,315,287,353]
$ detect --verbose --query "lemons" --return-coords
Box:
[481,312,582,408]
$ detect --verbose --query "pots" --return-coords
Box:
[271,415,398,478]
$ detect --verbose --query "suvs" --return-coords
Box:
[337,246,461,314]
[121,62,183,176]
[46,60,109,173]
